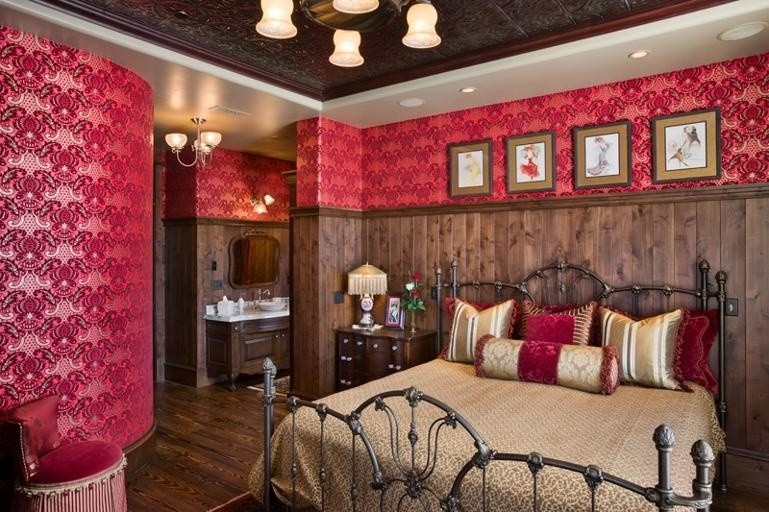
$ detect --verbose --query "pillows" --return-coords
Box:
[474,330,623,395]
[521,299,597,345]
[439,296,519,370]
[676,306,723,390]
[597,306,694,395]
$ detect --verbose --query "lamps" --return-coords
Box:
[344,258,386,333]
[252,0,443,70]
[166,116,221,170]
[249,190,276,218]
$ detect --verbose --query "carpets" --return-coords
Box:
[245,373,289,397]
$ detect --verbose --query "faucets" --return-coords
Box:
[256,287,271,302]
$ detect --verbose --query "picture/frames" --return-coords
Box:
[384,296,403,330]
[506,128,555,193]
[650,110,721,183]
[569,119,631,189]
[445,139,493,197]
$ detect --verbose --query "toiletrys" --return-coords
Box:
[238,295,244,312]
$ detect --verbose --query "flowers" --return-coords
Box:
[404,273,426,312]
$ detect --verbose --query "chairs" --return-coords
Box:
[1,393,131,511]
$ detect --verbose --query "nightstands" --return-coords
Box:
[336,323,436,387]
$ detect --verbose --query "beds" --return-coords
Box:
[267,249,739,511]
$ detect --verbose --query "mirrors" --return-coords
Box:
[227,230,281,289]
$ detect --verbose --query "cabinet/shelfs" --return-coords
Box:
[233,319,291,374]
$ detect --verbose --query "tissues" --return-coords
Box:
[216,295,235,315]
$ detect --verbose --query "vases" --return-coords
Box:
[410,314,420,331]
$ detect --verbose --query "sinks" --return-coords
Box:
[256,300,287,311]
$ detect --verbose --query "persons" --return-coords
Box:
[587,137,619,176]
[390,301,398,322]
[519,147,541,179]
